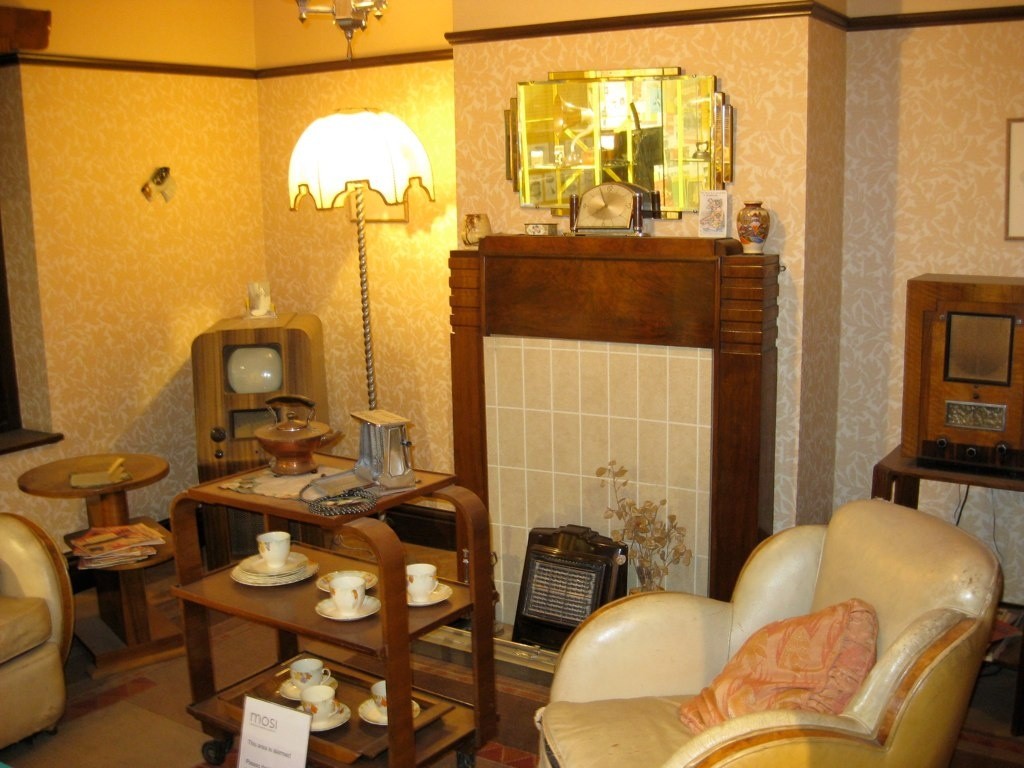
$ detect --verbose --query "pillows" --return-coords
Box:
[677,598,881,732]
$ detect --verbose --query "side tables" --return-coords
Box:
[17,452,187,681]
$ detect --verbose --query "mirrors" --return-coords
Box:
[505,69,734,219]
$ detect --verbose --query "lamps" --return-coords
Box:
[287,109,434,522]
[296,0,389,62]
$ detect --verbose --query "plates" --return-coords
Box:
[315,595,381,621]
[407,583,453,607]
[231,552,318,586]
[297,703,351,731]
[316,570,379,592]
[279,675,338,700]
[359,697,420,725]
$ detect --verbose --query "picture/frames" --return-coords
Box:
[349,180,410,225]
[1005,117,1024,242]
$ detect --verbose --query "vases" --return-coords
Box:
[736,200,769,254]
[461,214,493,249]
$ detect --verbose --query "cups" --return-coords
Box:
[462,215,491,250]
[290,658,331,690]
[301,685,339,722]
[406,563,439,602]
[256,531,291,569]
[329,577,368,613]
[371,680,388,716]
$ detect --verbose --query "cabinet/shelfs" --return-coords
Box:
[172,452,501,768]
[191,310,331,566]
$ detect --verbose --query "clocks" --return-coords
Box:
[568,181,645,236]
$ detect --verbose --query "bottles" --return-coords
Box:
[737,201,770,255]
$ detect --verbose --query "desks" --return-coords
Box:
[872,446,1023,737]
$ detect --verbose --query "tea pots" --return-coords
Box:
[255,393,344,475]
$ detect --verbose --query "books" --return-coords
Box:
[70,523,166,569]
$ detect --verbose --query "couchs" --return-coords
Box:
[532,498,1004,767]
[0,511,76,752]
